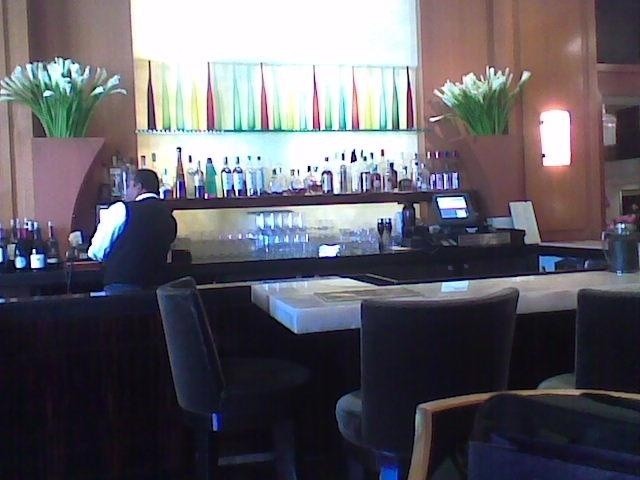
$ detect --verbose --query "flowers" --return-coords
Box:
[428,64,531,135]
[0,54,128,138]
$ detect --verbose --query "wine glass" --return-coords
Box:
[201,210,378,257]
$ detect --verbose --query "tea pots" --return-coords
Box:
[601,224,640,273]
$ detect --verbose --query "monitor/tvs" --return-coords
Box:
[432,193,477,226]
[596,0,640,64]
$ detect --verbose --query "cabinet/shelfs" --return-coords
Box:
[133,60,476,258]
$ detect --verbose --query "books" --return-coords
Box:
[314,287,424,304]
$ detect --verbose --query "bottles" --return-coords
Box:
[233,156,246,197]
[0,217,60,273]
[194,161,205,200]
[175,149,187,198]
[110,145,175,200]
[220,156,233,199]
[205,157,219,199]
[185,156,197,200]
[247,147,461,199]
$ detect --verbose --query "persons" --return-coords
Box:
[88,169,177,294]
